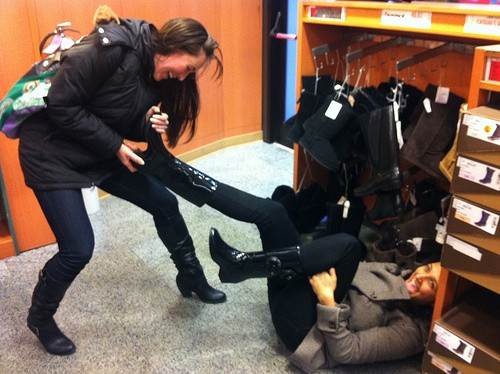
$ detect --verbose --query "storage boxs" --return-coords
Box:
[420,106,500,374]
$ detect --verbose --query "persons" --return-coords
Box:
[131,110,442,370]
[17,16,227,357]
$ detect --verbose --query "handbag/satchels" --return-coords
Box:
[0,53,63,138]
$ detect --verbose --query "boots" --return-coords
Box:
[157,215,227,302]
[132,111,219,207]
[26,268,77,356]
[209,228,301,282]
